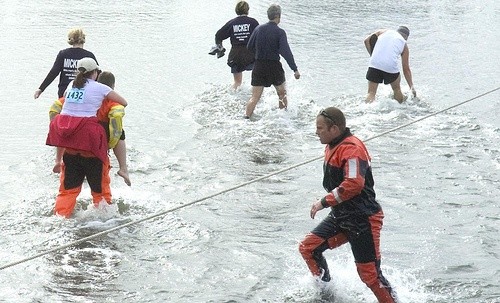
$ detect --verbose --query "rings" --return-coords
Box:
[311,209,315,212]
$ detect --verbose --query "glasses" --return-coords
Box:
[319,108,336,123]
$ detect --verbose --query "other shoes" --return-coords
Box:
[208,45,226,58]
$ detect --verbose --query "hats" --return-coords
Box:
[77,57,100,74]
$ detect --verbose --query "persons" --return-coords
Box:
[215,1,260,90]
[33,28,132,219]
[244,4,300,118]
[299,106,399,303]
[363,26,417,103]
